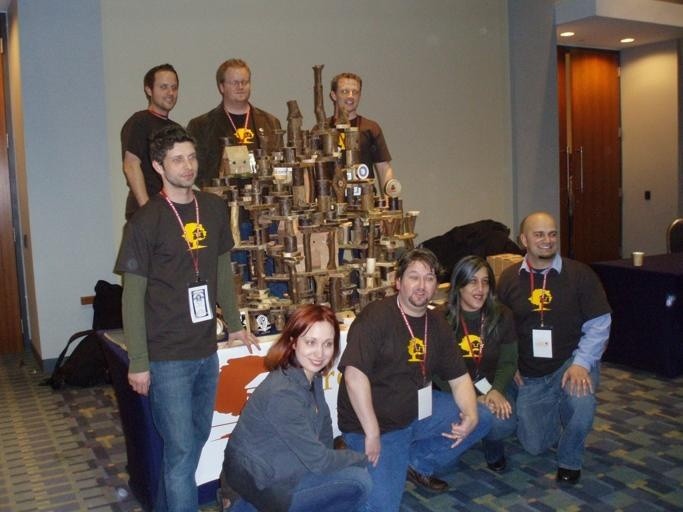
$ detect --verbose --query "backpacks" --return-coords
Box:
[46,329,112,391]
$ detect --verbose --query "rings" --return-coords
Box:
[456,435,458,440]
[582,379,586,383]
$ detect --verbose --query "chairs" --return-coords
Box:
[664,216,682,254]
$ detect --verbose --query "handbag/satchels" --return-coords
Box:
[91,279,124,331]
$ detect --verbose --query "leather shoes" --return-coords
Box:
[556,465,582,485]
[406,465,448,493]
[483,439,506,472]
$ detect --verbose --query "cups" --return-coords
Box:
[631,251,645,267]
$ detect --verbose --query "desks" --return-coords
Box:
[88,283,452,511]
[587,248,681,380]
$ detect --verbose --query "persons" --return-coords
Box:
[186,57,293,297]
[215,304,374,512]
[335,248,496,512]
[112,126,261,512]
[494,211,614,486]
[120,62,188,221]
[310,73,394,209]
[438,253,519,474]
